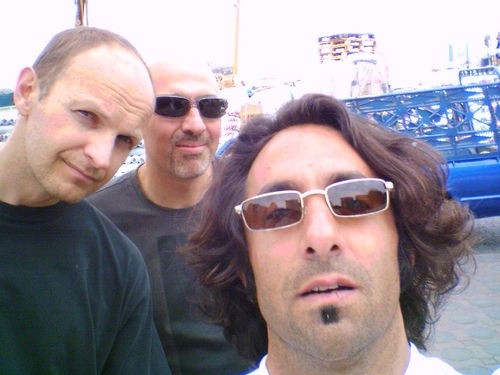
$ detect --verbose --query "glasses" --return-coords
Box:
[233,177,394,233]
[155,96,229,118]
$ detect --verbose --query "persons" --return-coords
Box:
[179,90,481,375]
[0,24,172,375]
[84,48,259,374]
